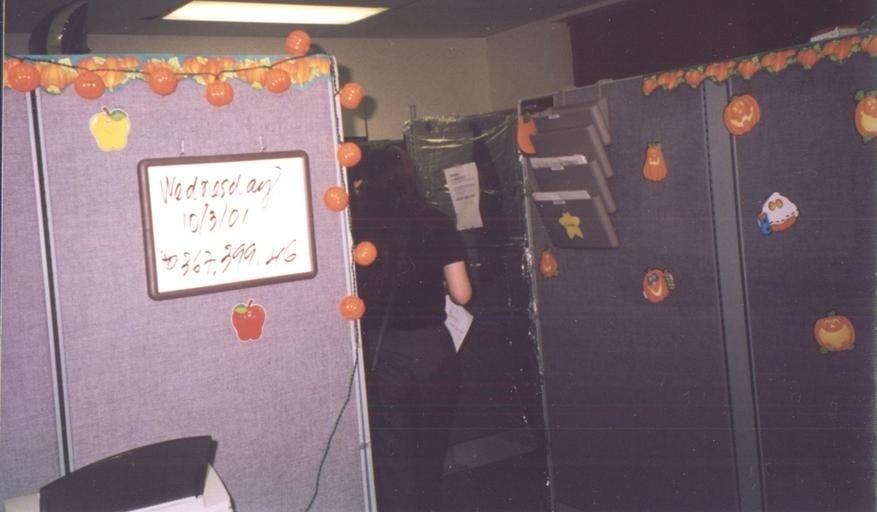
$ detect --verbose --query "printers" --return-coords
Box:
[0,434,235,512]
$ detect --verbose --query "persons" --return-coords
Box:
[352,144,472,511]
